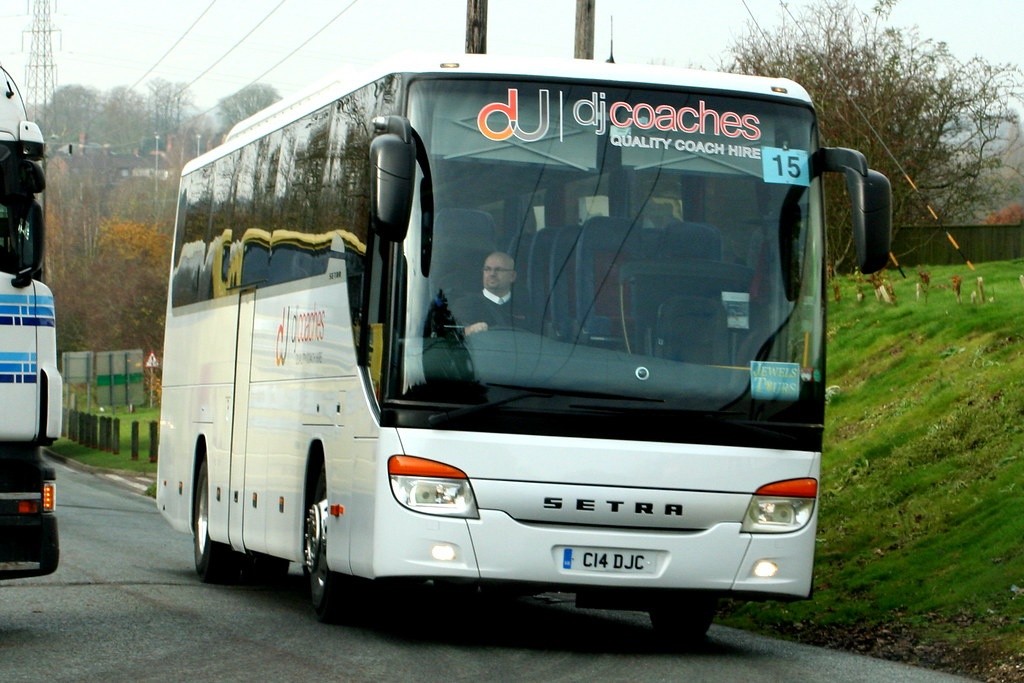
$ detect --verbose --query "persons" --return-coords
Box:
[453,249,550,333]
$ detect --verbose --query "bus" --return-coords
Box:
[155,50,890,646]
[0,65,66,580]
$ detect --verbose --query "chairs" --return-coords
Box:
[431,206,725,358]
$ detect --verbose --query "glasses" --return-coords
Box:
[481,268,513,275]
